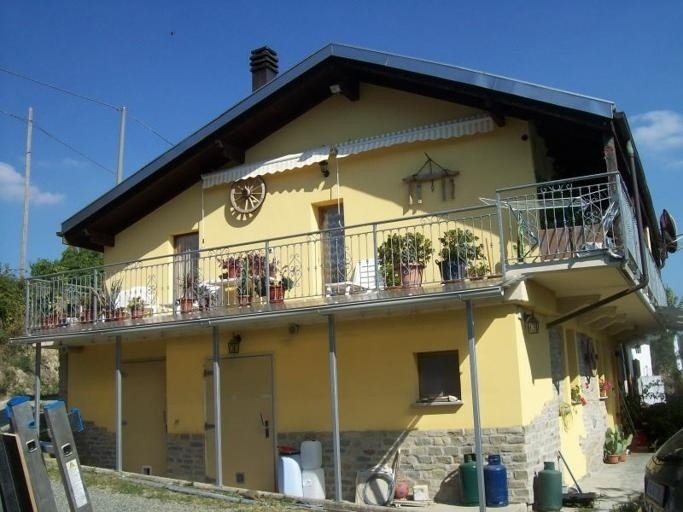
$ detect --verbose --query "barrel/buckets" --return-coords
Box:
[301,468,326,500]
[278,455,302,499]
[300,440,323,469]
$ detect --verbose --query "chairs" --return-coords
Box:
[324,259,376,298]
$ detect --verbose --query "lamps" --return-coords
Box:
[630,343,641,354]
[318,160,330,177]
[228,334,242,355]
[525,308,538,335]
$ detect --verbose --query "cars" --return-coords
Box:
[641,427,682,512]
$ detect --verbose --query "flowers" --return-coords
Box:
[600,381,614,389]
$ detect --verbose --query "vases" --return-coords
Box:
[601,390,606,396]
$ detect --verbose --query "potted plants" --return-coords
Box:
[375,232,433,287]
[178,271,215,314]
[217,250,294,308]
[433,229,491,283]
[41,279,145,328]
[605,424,634,463]
